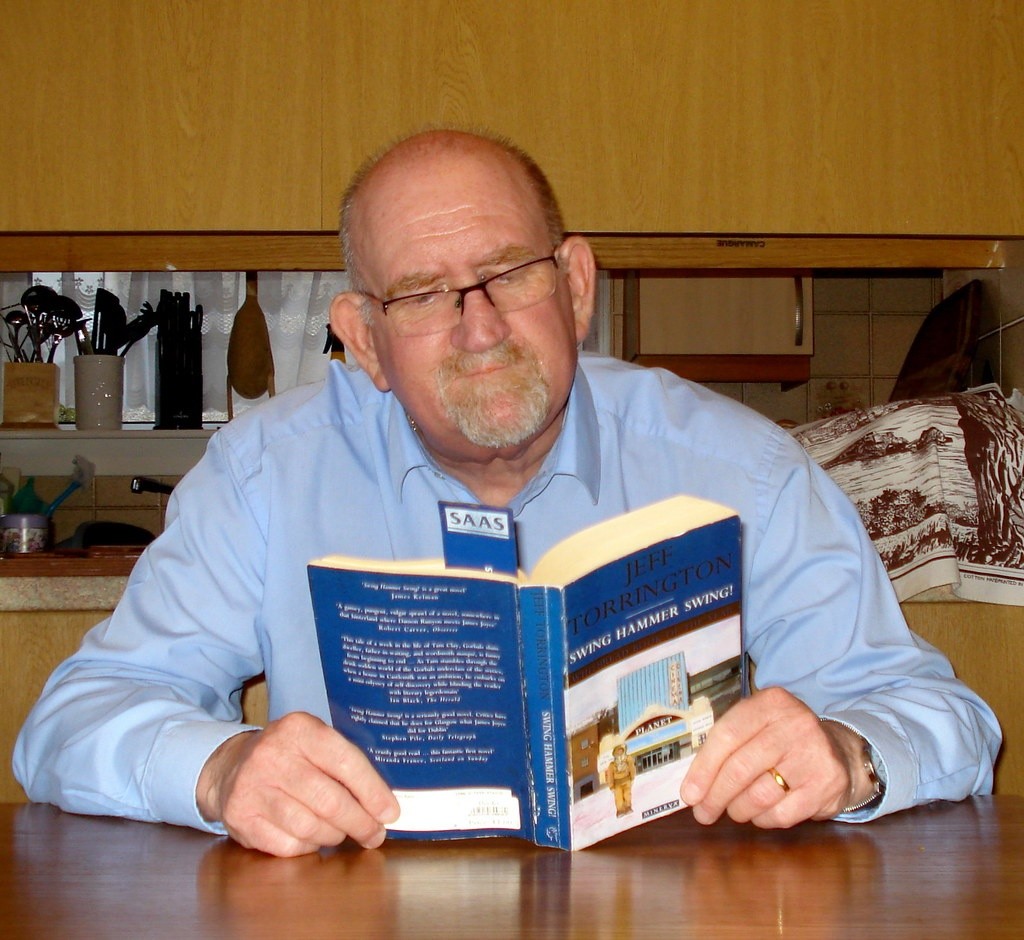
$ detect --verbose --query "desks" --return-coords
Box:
[0,791,1023,940]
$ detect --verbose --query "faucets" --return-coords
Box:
[129,477,173,495]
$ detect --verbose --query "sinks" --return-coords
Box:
[82,546,146,555]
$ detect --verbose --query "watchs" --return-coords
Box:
[820,718,887,813]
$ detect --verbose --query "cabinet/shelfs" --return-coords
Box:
[620,268,816,393]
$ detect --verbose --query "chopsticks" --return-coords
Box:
[156,289,204,339]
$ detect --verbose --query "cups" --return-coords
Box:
[155,338,204,430]
[72,355,125,430]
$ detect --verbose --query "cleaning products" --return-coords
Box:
[322,323,346,365]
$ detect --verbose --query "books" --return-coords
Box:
[307,495,745,853]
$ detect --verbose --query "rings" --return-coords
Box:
[768,768,792,795]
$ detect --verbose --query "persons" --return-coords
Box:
[11,130,1003,858]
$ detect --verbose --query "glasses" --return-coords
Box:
[353,242,558,337]
